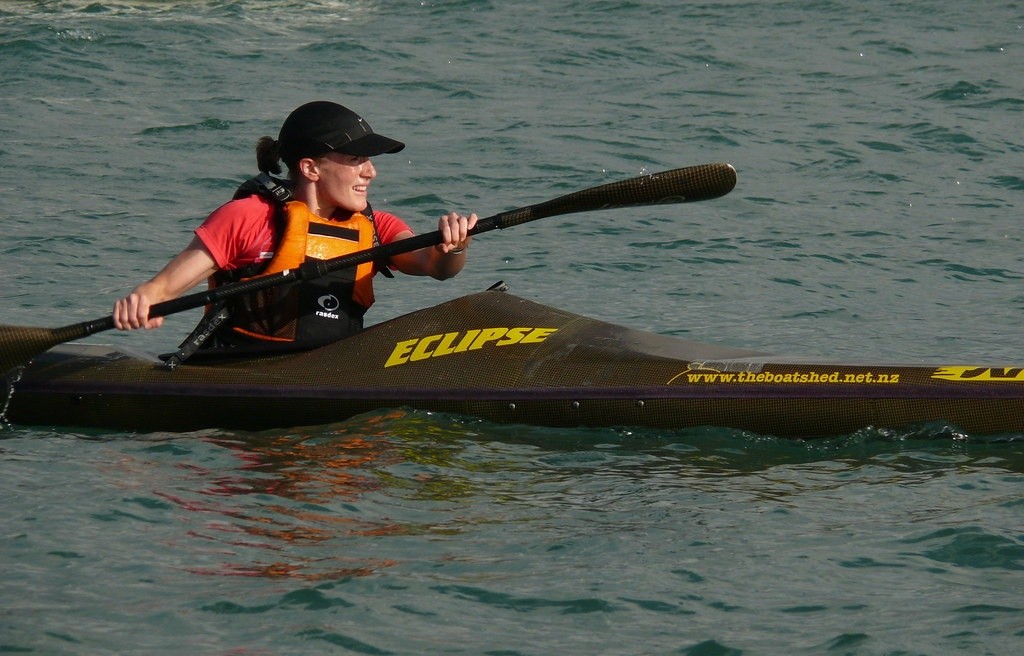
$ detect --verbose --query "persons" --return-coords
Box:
[114,99,478,366]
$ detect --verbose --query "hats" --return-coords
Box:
[278,100,406,166]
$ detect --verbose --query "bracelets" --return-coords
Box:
[450,247,464,254]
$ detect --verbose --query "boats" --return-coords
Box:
[0,279,1023,433]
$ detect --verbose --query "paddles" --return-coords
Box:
[0,159,739,376]
